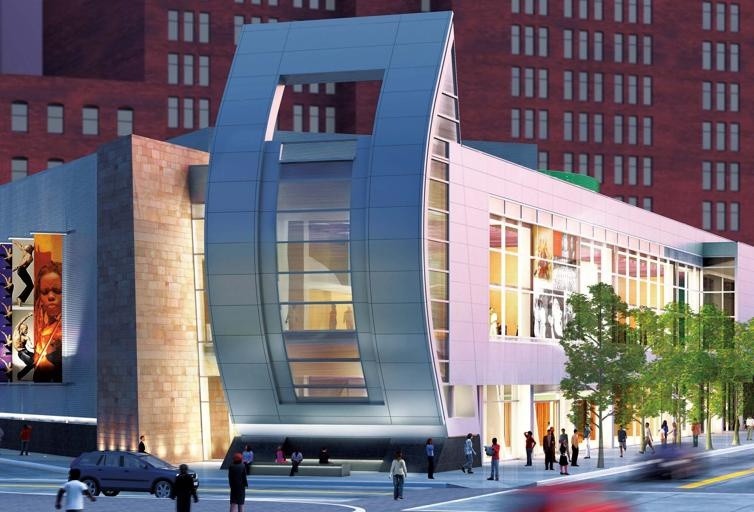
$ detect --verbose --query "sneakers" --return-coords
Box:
[487,478,494,480]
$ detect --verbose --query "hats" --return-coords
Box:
[233,453,242,461]
